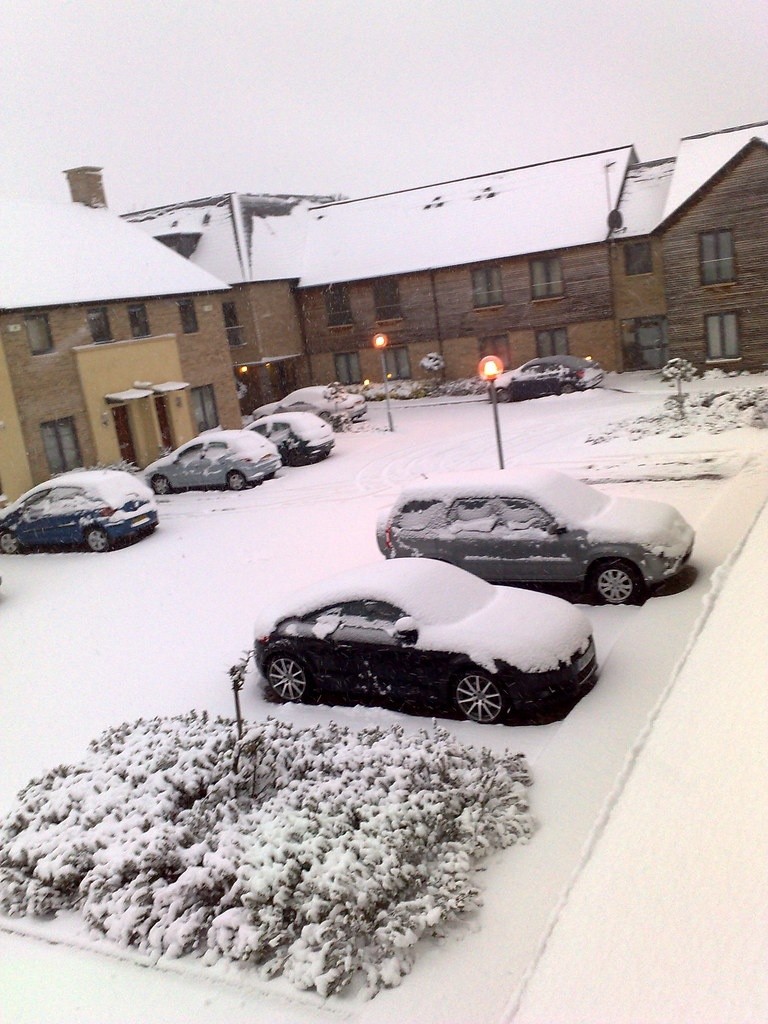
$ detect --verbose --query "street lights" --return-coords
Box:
[372,333,395,432]
[478,356,505,470]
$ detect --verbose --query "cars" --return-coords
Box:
[252,385,367,432]
[0,471,160,554]
[254,557,599,724]
[243,411,336,467]
[374,467,698,606]
[144,430,282,495]
[487,354,605,403]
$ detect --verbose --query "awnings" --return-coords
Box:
[104,388,154,409]
[151,381,189,399]
